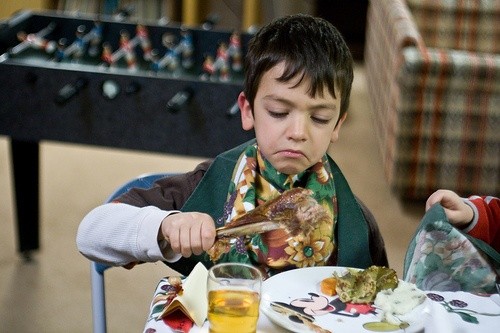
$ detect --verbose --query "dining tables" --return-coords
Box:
[142,274,500,333]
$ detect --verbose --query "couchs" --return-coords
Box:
[361,0,500,213]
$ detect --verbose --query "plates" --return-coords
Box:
[263,267,433,333]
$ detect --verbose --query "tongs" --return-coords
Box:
[215,197,317,240]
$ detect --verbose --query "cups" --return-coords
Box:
[206,263,262,333]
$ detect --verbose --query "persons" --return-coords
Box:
[74,15,393,281]
[424,189,500,253]
